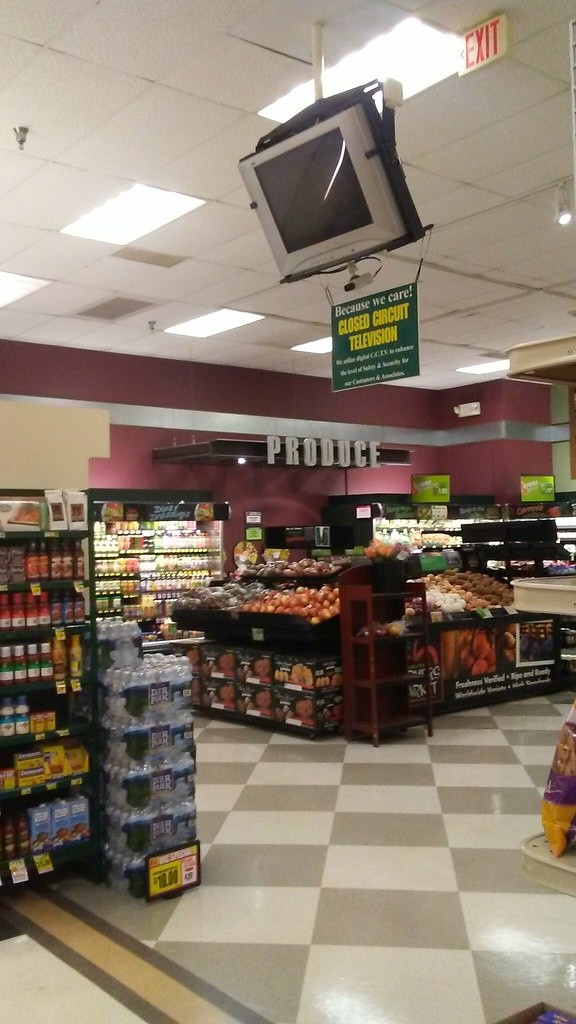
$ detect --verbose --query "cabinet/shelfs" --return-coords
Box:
[0,488,107,894]
[176,555,390,740]
[339,561,433,748]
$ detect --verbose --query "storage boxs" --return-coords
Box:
[174,643,343,729]
[0,737,89,791]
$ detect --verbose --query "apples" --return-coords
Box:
[358,620,401,638]
[240,585,339,626]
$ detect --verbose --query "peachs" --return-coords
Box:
[459,632,498,675]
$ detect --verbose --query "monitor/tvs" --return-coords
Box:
[238,102,407,277]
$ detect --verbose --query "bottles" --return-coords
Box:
[0,537,88,856]
[94,530,221,624]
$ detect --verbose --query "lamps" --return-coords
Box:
[454,402,481,417]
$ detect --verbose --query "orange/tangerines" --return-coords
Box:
[363,546,391,557]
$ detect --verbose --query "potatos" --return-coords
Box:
[273,558,343,574]
[243,563,281,575]
[179,583,269,608]
[413,567,513,611]
